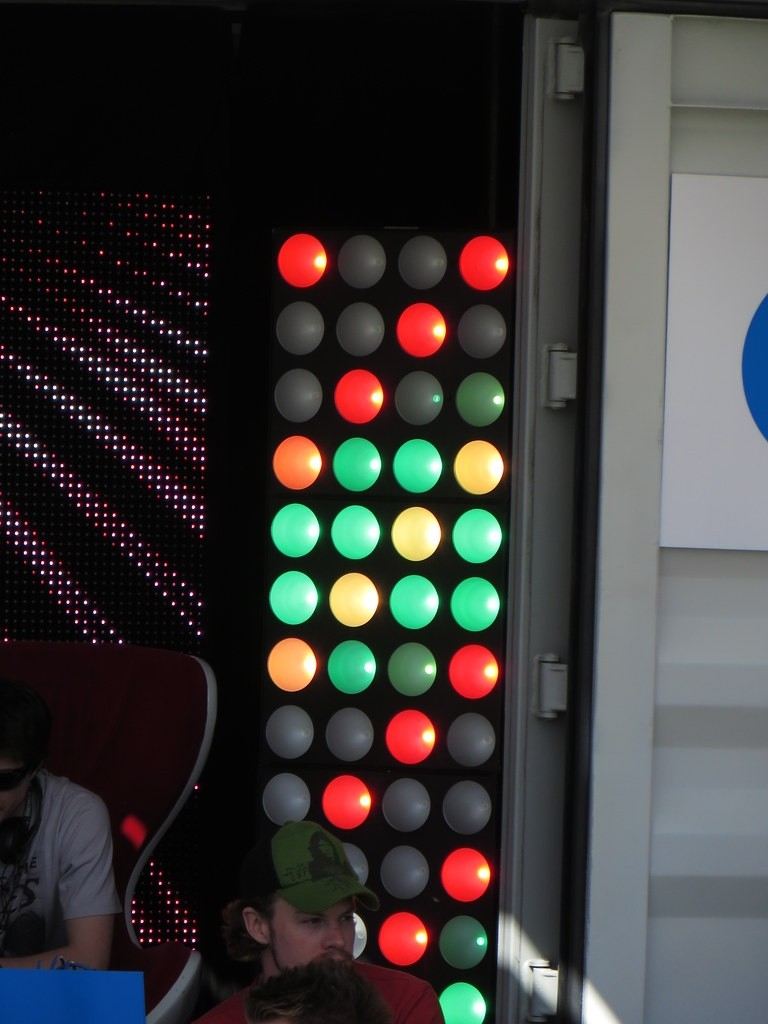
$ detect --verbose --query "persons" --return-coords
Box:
[244,957,394,1024]
[0,721,124,970]
[196,820,447,1024]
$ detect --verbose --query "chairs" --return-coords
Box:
[0,641,218,1024]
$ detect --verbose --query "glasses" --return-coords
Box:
[0,765,31,791]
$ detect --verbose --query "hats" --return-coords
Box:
[245,820,379,910]
